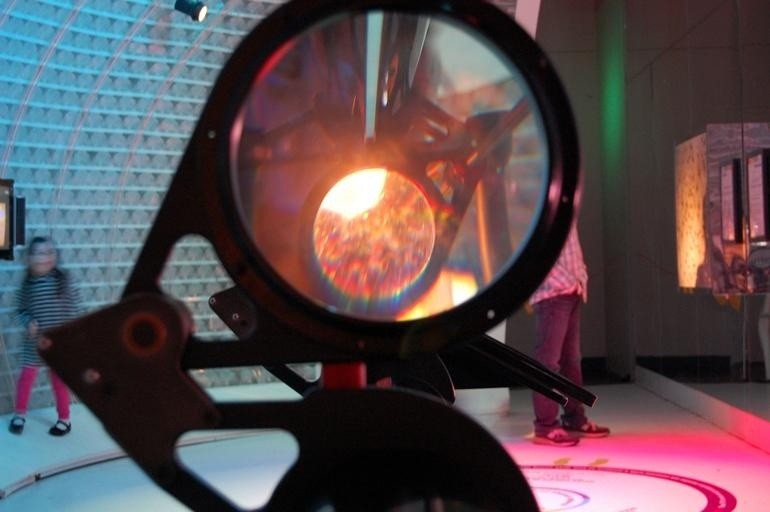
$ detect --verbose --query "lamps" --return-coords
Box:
[175,0,208,22]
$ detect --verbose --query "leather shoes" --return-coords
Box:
[8,417,26,435]
[47,419,73,436]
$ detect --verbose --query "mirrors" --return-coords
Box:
[35,0,579,511]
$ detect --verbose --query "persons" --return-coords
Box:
[9,236,86,436]
[522,224,610,446]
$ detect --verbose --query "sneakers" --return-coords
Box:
[560,412,611,439]
[530,418,579,448]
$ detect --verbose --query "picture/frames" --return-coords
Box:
[718,158,744,244]
[746,149,770,242]
